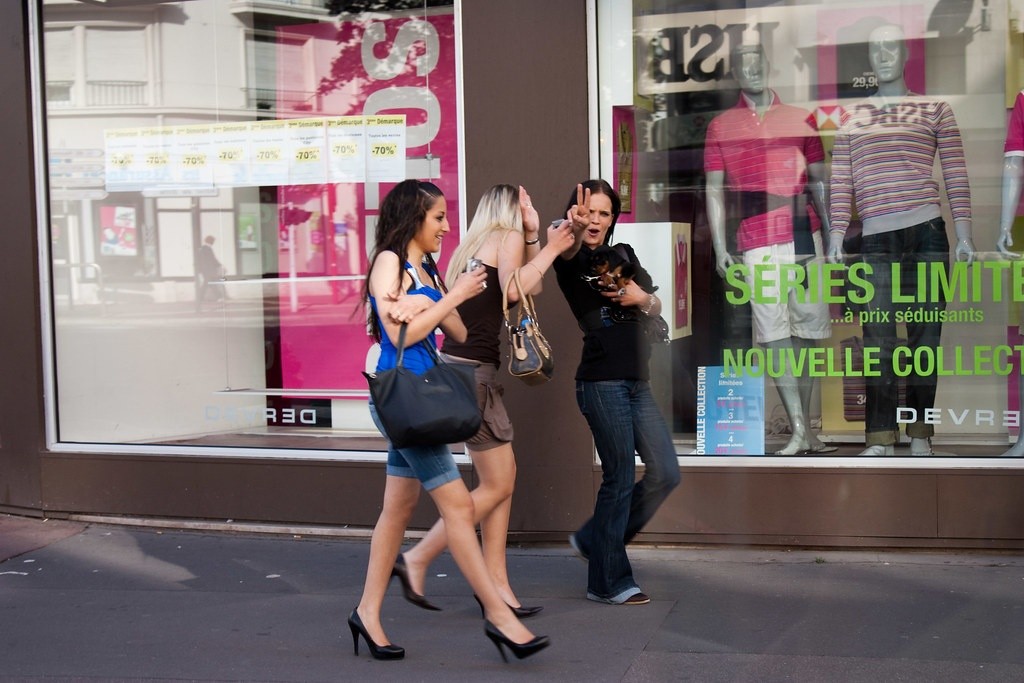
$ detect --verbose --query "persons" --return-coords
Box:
[195,235,229,312]
[348,180,552,662]
[317,215,349,303]
[552,179,682,606]
[704,24,1024,457]
[390,184,576,618]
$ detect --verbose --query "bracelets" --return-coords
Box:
[525,238,539,245]
[527,262,544,280]
[641,294,655,315]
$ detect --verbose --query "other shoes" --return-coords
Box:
[622,593,650,604]
[569,534,589,563]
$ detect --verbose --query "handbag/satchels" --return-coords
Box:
[361,321,481,450]
[611,243,671,346]
[503,267,554,387]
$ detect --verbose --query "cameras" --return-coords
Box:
[552,219,565,229]
[467,259,481,275]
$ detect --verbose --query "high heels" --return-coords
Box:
[473,593,544,620]
[388,553,441,611]
[483,619,550,663]
[348,607,405,660]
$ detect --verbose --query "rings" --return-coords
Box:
[526,205,531,208]
[396,310,400,316]
[481,282,487,290]
[570,233,575,239]
[617,287,626,296]
